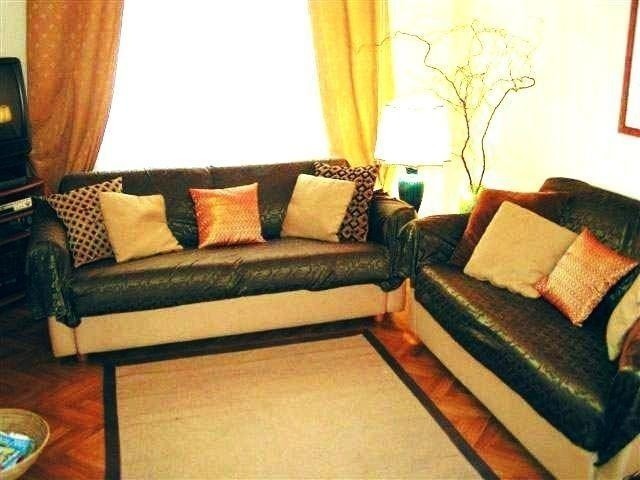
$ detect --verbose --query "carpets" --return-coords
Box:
[101,326,500,480]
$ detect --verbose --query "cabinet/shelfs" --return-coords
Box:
[0,179,47,306]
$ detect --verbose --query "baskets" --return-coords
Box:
[0,408,50,480]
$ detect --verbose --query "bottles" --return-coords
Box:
[397,166,424,214]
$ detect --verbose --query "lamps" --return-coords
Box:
[373,103,456,211]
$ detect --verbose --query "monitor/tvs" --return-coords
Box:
[0,57,32,160]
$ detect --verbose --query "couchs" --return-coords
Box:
[23,157,418,363]
[396,175,640,480]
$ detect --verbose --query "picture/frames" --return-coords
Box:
[617,0,640,138]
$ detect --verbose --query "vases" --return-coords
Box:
[460,179,486,214]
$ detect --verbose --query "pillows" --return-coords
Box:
[94,189,183,265]
[45,176,125,268]
[446,186,640,374]
[279,174,356,244]
[310,161,384,243]
[187,182,267,249]
